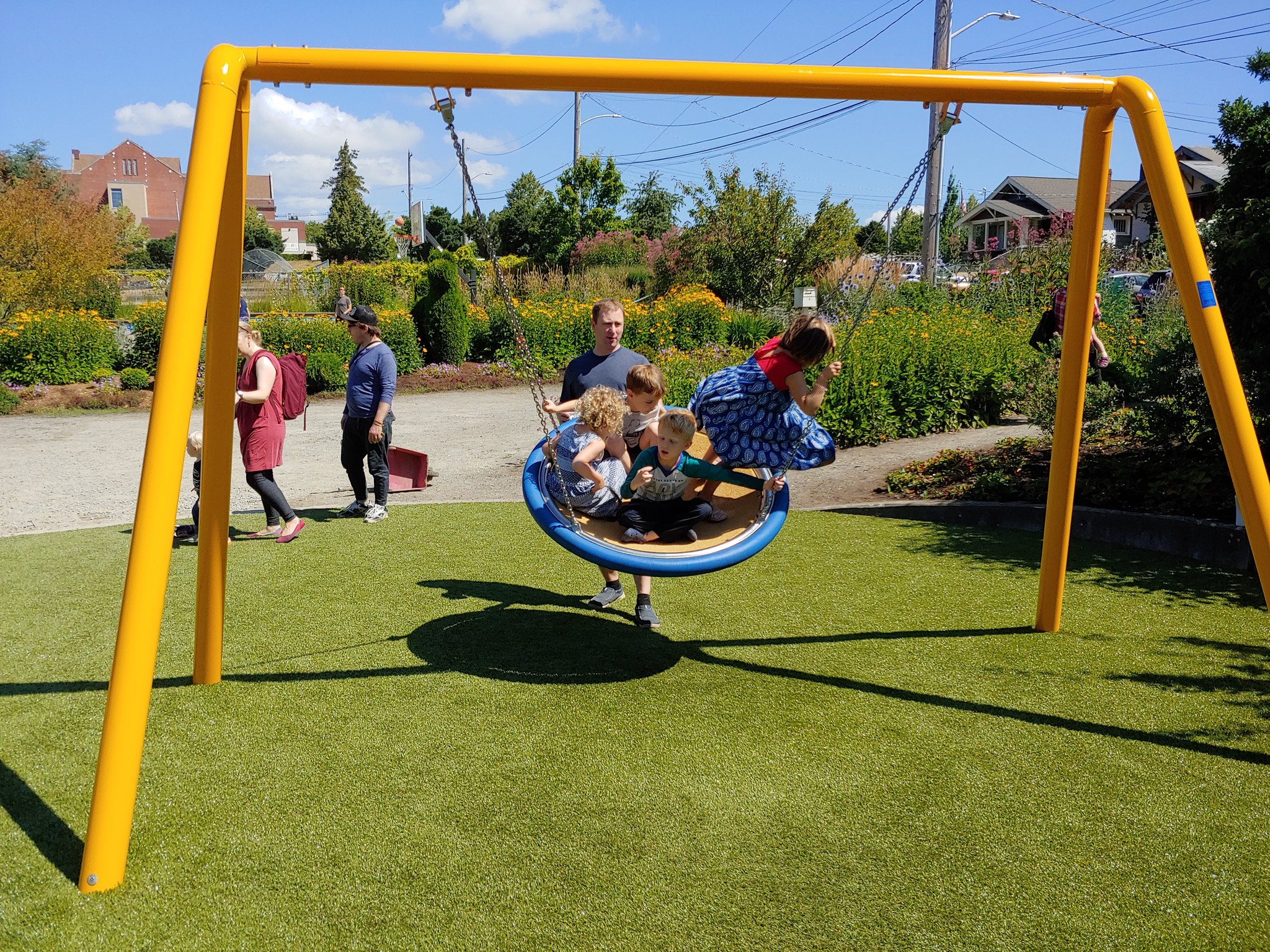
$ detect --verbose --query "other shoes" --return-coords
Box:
[188,534,198,543]
[1098,355,1111,367]
[276,519,306,543]
[706,501,728,522]
[247,526,283,537]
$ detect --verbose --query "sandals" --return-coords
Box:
[684,529,698,541]
[620,528,647,545]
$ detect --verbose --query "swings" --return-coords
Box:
[435,100,958,577]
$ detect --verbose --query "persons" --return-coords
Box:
[541,384,630,519]
[559,298,665,627]
[542,364,667,474]
[336,305,396,523]
[239,294,251,322]
[186,431,204,542]
[686,315,841,524]
[235,320,305,543]
[1091,292,1110,368]
[1053,271,1104,390]
[621,409,786,542]
[334,286,352,326]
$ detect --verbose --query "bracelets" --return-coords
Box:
[373,422,383,428]
[374,418,383,426]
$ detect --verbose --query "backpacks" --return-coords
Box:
[254,349,309,432]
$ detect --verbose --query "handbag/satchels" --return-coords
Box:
[1028,288,1060,359]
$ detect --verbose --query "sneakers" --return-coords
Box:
[588,583,625,610]
[363,503,388,525]
[635,603,661,628]
[336,500,373,517]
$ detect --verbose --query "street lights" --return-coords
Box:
[933,9,1022,294]
[172,190,180,223]
[401,186,434,218]
[578,113,622,157]
[462,171,495,246]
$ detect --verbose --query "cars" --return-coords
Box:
[898,261,970,284]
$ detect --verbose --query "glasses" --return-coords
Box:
[339,289,345,291]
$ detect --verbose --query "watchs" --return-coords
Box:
[236,390,243,400]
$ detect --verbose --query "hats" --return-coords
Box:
[339,304,378,326]
